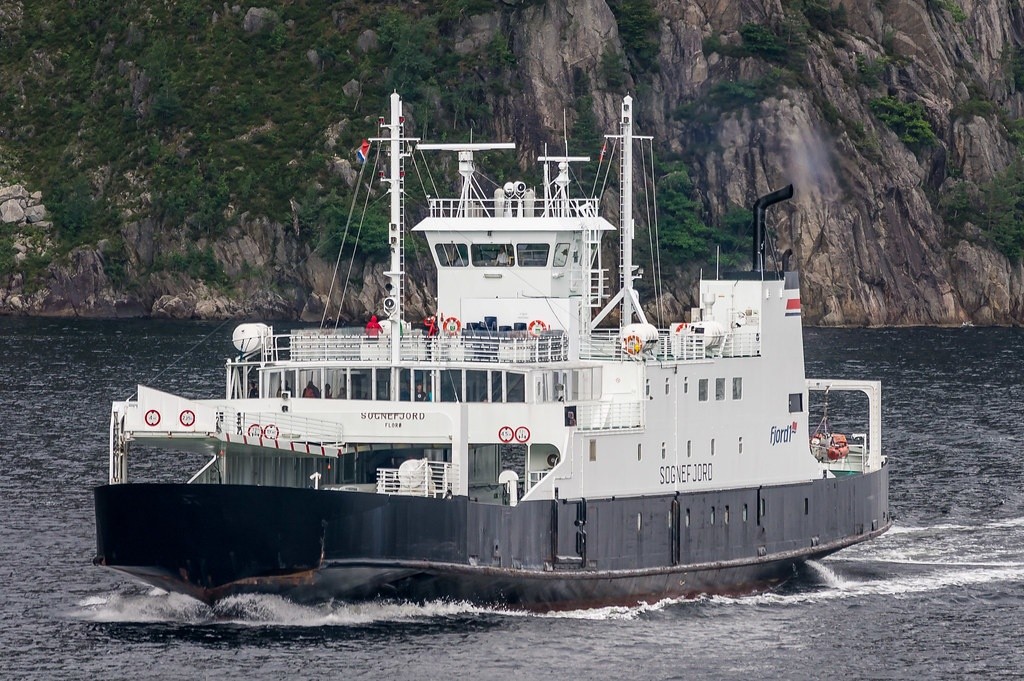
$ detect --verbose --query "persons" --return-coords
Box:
[495,247,508,266]
[247,380,346,398]
[365,315,383,341]
[377,384,432,401]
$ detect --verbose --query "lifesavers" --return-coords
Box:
[624,334,643,355]
[442,316,461,336]
[528,320,547,339]
[675,323,694,338]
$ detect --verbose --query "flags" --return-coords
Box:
[356,139,371,162]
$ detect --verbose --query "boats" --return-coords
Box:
[92,87,893,626]
